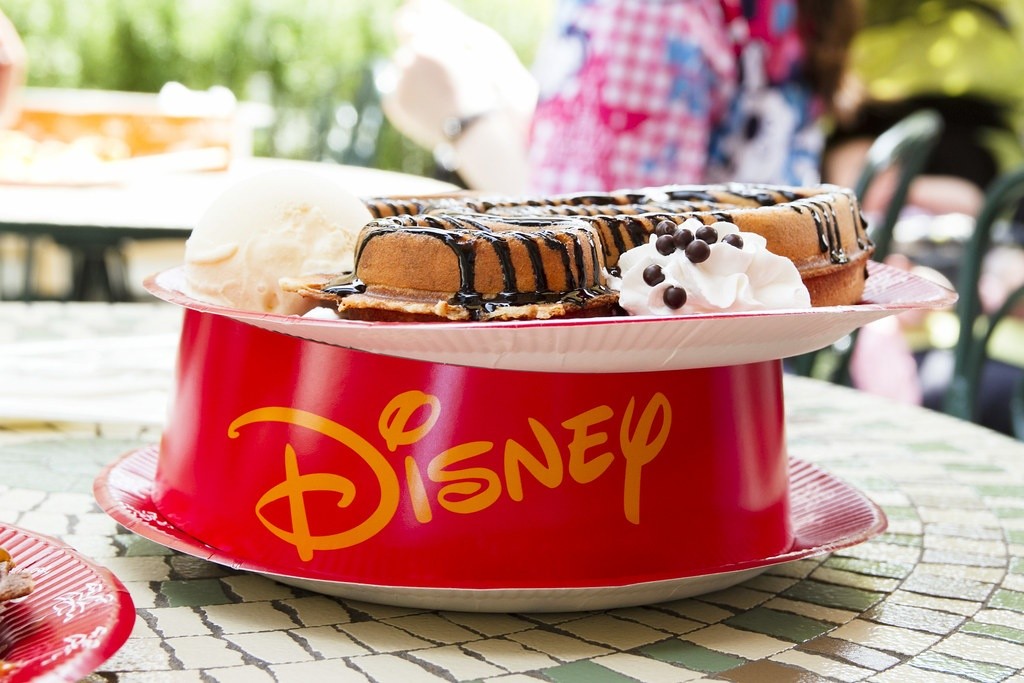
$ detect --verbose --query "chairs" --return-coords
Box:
[950,161,1024,444]
[802,108,939,387]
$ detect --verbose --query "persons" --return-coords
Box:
[374,0,800,262]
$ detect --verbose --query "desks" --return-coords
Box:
[0,298,1024,683]
[0,153,464,304]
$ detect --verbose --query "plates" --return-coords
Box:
[94,443,884,612]
[0,522,135,683]
[145,270,960,373]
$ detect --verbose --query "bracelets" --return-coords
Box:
[436,109,484,144]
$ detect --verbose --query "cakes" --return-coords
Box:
[184,172,874,327]
